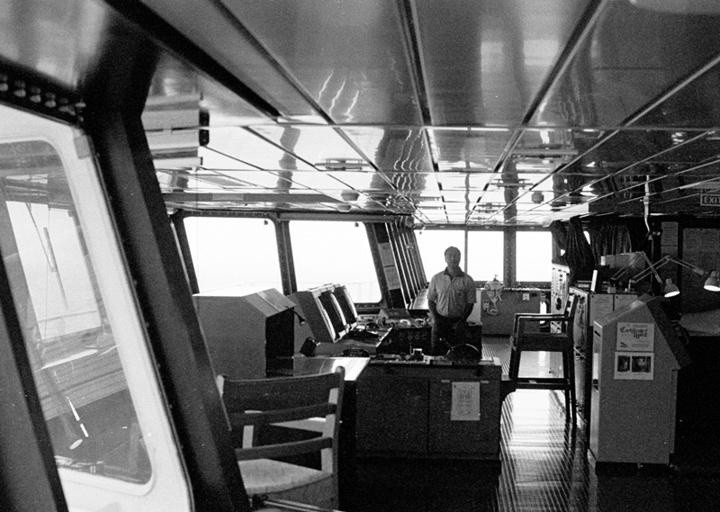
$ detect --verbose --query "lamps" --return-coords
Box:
[608,250,719,298]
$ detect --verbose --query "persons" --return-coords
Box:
[426,246,478,355]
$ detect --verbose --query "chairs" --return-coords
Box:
[212,365,345,511]
[509,293,573,429]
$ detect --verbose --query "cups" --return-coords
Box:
[413,348,423,360]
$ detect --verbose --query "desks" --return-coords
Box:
[366,354,429,461]
[429,356,501,461]
